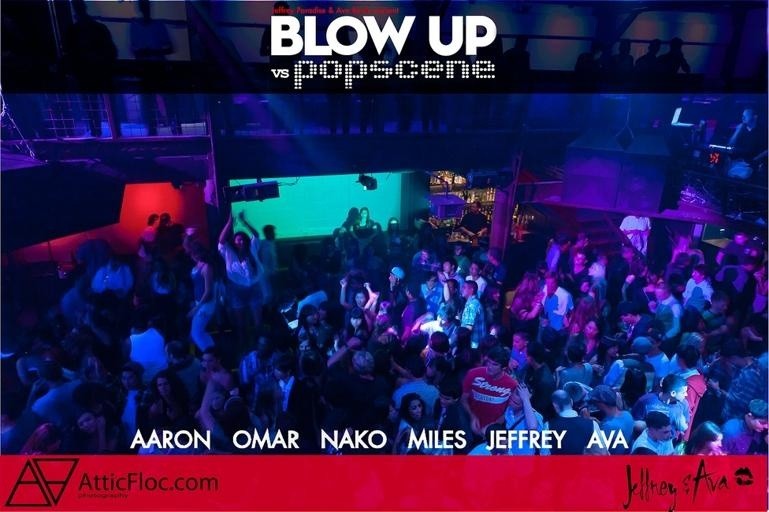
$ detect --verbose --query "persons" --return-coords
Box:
[635,38,661,72]
[60,0,122,138]
[610,38,634,75]
[128,1,185,136]
[188,11,248,136]
[259,1,309,135]
[728,104,768,163]
[657,36,691,74]
[575,38,604,72]
[1,202,768,456]
[324,15,532,136]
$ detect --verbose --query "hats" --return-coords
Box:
[391,266,406,281]
[588,384,616,403]
